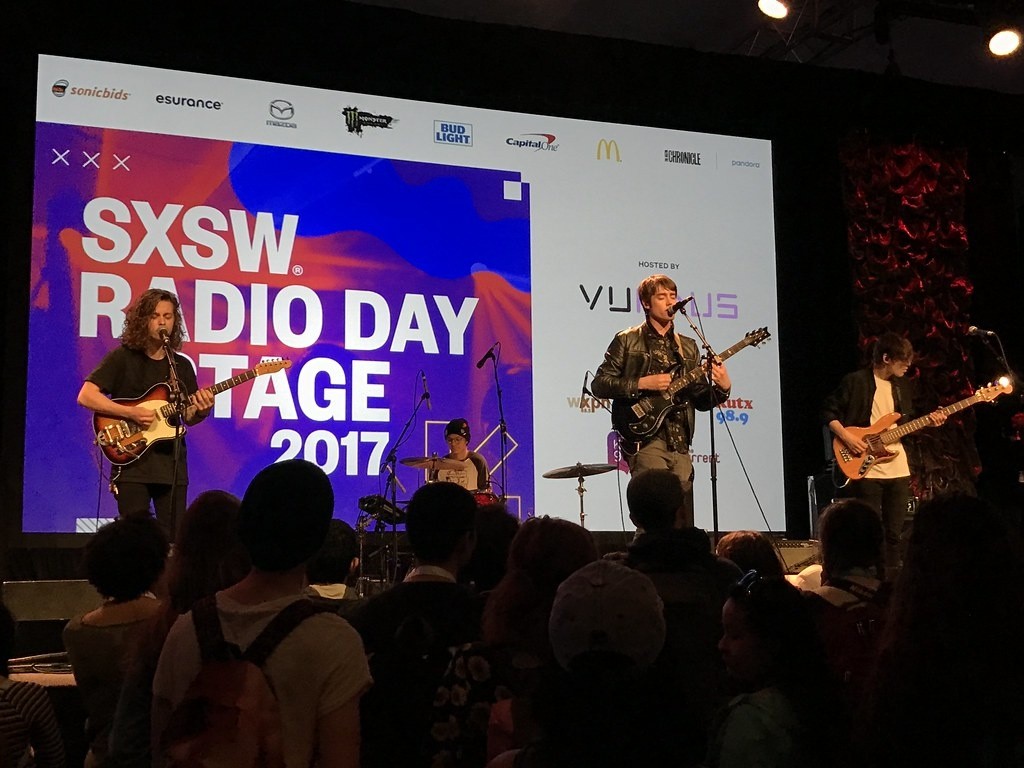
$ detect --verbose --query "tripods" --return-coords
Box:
[353,511,384,604]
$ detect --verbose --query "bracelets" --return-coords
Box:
[195,410,210,419]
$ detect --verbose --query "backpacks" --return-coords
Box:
[158,594,341,767]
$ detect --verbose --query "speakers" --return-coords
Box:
[0,579,112,666]
[772,539,823,576]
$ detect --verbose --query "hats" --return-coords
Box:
[239,460,334,572]
[548,559,667,676]
[444,418,471,445]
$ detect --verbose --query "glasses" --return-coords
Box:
[447,437,463,444]
[737,569,794,601]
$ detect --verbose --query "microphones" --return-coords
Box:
[968,325,995,336]
[578,372,589,408]
[477,343,498,368]
[422,372,432,411]
[158,327,170,344]
[668,296,693,315]
[385,464,406,495]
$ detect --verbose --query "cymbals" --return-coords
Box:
[542,463,618,478]
[399,455,466,472]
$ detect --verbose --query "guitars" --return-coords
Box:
[92,356,293,467]
[832,373,1018,481]
[628,327,771,444]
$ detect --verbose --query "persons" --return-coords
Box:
[434,418,492,493]
[0,458,1024,768]
[823,332,947,575]
[591,275,732,543]
[76,289,215,546]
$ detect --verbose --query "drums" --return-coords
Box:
[469,487,500,506]
